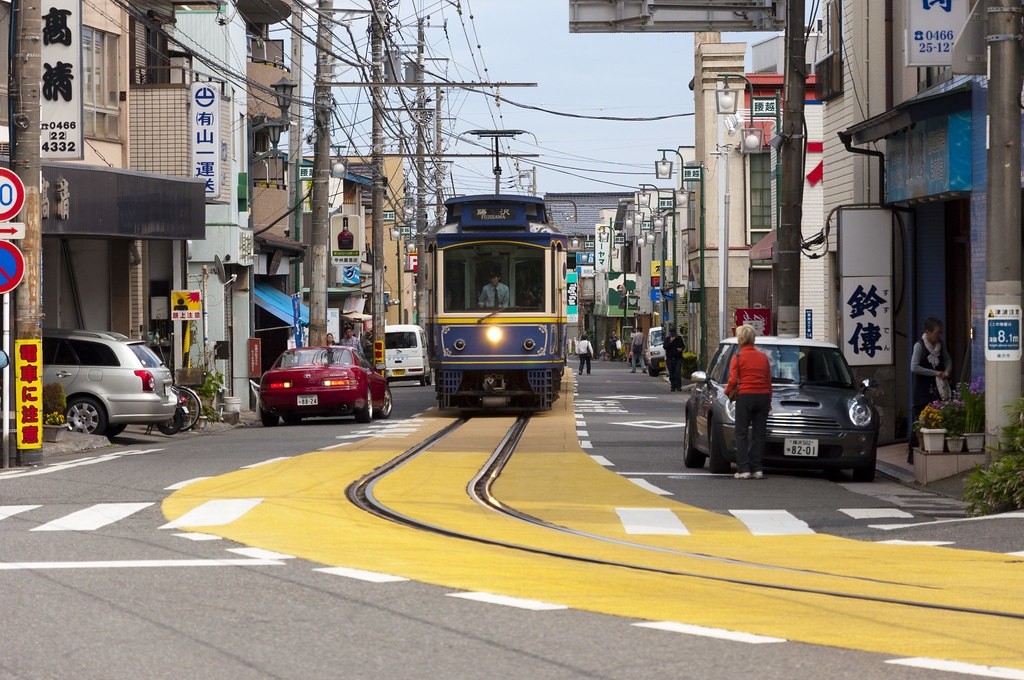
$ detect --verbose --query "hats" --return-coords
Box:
[669,329,676,332]
[636,326,642,329]
[489,272,499,278]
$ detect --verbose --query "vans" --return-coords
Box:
[385,324,432,386]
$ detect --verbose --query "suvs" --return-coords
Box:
[42,325,178,437]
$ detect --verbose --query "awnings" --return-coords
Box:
[252,280,310,331]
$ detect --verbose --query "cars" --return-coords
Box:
[685,332,881,482]
[259,349,397,428]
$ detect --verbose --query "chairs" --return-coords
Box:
[250,380,261,420]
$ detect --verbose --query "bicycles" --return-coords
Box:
[179,386,202,432]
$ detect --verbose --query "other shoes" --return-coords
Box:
[670,389,675,391]
[642,369,647,373]
[677,389,682,392]
[728,389,738,401]
[579,371,582,374]
[751,471,763,479]
[734,472,751,478]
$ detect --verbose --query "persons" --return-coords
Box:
[663,329,685,392]
[326,326,373,369]
[630,326,647,373]
[912,316,951,417]
[578,334,594,375]
[724,325,773,479]
[608,329,619,362]
[478,269,510,307]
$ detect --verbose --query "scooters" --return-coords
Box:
[146,387,190,435]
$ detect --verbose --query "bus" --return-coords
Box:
[427,193,567,414]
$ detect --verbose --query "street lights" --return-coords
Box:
[625,209,655,312]
[390,196,415,324]
[654,148,707,370]
[634,203,666,327]
[716,71,780,305]
[246,75,298,412]
[599,227,626,341]
[570,231,596,359]
[638,183,677,329]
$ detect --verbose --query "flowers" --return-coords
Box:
[912,377,986,439]
[43,382,67,424]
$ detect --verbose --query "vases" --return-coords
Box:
[945,437,965,452]
[916,434,924,450]
[961,432,985,452]
[43,423,67,442]
[920,428,947,453]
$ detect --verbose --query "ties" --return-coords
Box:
[494,289,498,307]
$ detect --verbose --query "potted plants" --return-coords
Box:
[193,364,225,428]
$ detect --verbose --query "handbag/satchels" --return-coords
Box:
[634,344,642,353]
[936,374,952,400]
[669,358,677,368]
[616,339,622,349]
[586,342,591,357]
